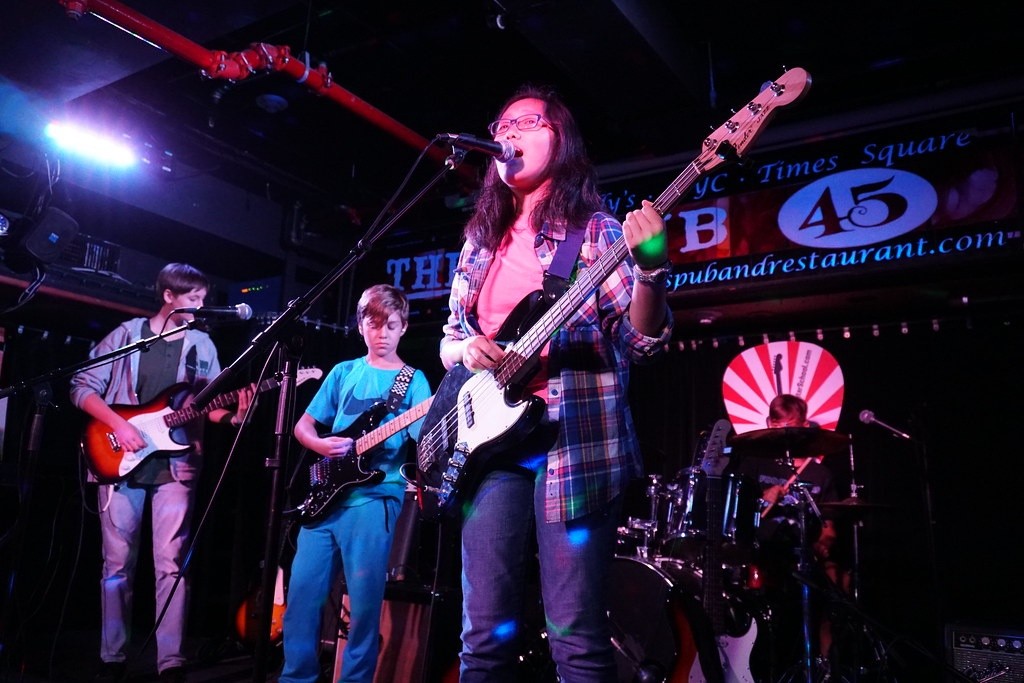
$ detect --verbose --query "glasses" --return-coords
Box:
[488,114,557,135]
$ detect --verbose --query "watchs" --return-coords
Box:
[632,262,672,287]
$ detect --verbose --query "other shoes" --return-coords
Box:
[95,654,127,683]
[159,667,187,683]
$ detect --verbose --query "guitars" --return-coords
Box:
[80,363,322,484]
[416,60,810,518]
[291,388,436,527]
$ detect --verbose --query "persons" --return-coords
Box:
[69,263,260,683]
[277,283,432,683]
[437,77,675,683]
[750,394,850,682]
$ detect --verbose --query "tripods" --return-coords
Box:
[769,443,907,683]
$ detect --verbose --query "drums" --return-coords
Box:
[609,509,699,683]
[651,465,747,571]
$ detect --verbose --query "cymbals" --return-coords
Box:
[816,498,894,513]
[724,422,851,461]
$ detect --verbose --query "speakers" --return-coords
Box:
[328,579,466,683]
[945,621,1024,683]
[20,206,79,264]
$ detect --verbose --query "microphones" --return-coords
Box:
[174,302,252,320]
[858,409,910,439]
[438,133,515,163]
